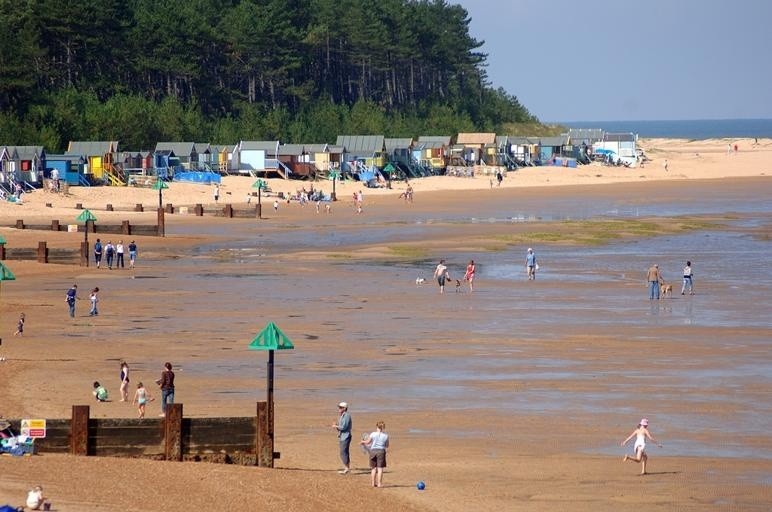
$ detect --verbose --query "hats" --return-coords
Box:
[338,402,348,409]
[640,419,649,426]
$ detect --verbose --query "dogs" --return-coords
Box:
[455,279,460,293]
[415,276,426,285]
[659,275,673,298]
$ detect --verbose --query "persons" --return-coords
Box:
[620,418,662,474]
[681,260,693,295]
[215,185,220,202]
[158,362,175,417]
[133,382,155,418]
[550,146,668,169]
[7,173,21,199]
[26,485,46,510]
[433,260,451,293]
[496,173,503,188]
[646,265,661,299]
[727,143,737,154]
[50,168,59,180]
[525,248,539,280]
[88,286,99,316]
[92,381,108,401]
[95,238,137,269]
[64,284,81,318]
[360,421,389,487]
[463,260,475,290]
[331,402,352,474]
[247,160,413,213]
[120,359,129,401]
[14,312,25,337]
